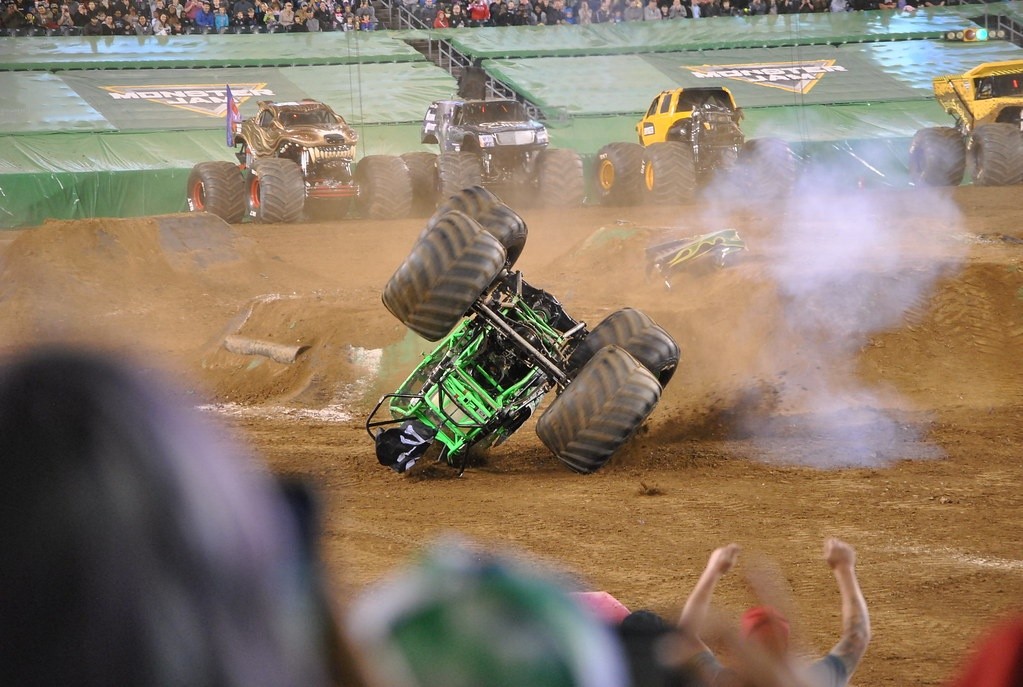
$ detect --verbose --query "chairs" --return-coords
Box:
[0,22,292,37]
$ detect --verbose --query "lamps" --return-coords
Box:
[944,27,1007,43]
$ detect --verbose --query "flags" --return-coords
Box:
[226,85,241,147]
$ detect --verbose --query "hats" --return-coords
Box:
[741,608,789,659]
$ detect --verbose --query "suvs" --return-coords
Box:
[591,87,796,209]
[186,98,412,224]
[908,60,1023,187]
[397,93,585,214]
[365,183,681,477]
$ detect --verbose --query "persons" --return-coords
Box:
[1,342,1022,687]
[0,0,946,36]
